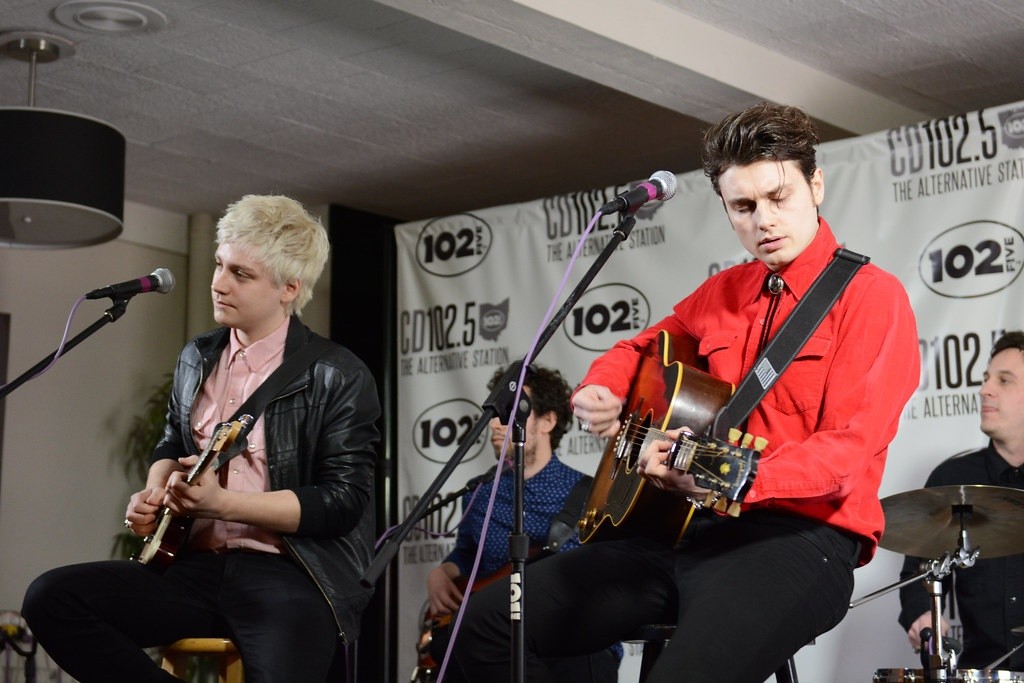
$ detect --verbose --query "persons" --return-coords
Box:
[421,357,625,683]
[17,192,386,683]
[896,331,1024,682]
[429,100,922,683]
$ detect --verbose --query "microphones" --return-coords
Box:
[600,170,676,215]
[86,268,176,299]
[466,456,515,492]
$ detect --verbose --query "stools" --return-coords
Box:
[624,624,791,683]
[160,637,245,683]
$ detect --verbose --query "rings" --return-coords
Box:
[580,420,590,432]
[123,519,134,530]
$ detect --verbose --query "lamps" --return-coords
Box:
[0,31,127,250]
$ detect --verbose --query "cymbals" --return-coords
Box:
[859,483,1024,563]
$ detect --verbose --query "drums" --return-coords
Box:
[873,665,1024,683]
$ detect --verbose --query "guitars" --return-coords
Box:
[127,412,257,568]
[576,326,768,543]
